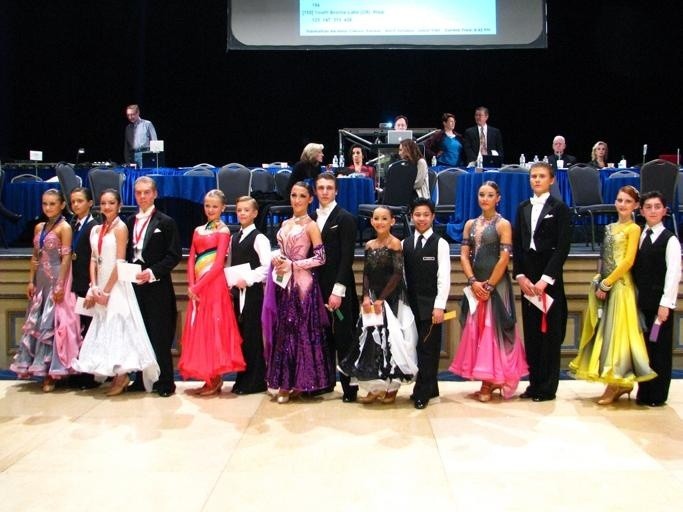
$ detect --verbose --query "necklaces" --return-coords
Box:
[290,212,309,225]
[476,213,495,226]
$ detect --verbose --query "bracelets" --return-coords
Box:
[27,280,33,283]
[55,278,68,287]
[597,278,613,292]
[482,282,496,292]
[467,276,477,284]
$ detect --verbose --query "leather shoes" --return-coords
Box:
[72,374,98,390]
[277,387,290,403]
[410,388,429,409]
[42,374,59,392]
[159,381,176,397]
[636,397,664,407]
[125,380,147,392]
[519,391,556,401]
[231,382,268,395]
[343,386,357,402]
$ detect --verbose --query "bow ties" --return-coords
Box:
[529,194,548,206]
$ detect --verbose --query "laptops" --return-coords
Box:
[388,130,413,143]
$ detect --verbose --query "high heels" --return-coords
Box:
[184,379,223,396]
[597,383,633,405]
[359,386,398,404]
[101,376,129,396]
[479,379,505,404]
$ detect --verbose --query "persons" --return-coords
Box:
[288,140,329,196]
[510,162,577,405]
[338,143,377,180]
[545,134,578,168]
[177,189,251,396]
[300,171,364,402]
[64,177,178,396]
[424,109,462,172]
[566,183,658,406]
[462,108,502,170]
[389,113,407,133]
[397,138,432,204]
[336,204,419,406]
[630,189,682,407]
[585,142,615,170]
[226,193,274,397]
[399,196,452,410]
[7,184,86,394]
[260,179,336,406]
[122,104,161,169]
[446,178,529,405]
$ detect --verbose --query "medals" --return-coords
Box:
[35,249,42,260]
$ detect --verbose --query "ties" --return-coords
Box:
[236,229,243,243]
[74,221,82,233]
[640,228,653,253]
[479,127,488,156]
[414,234,425,250]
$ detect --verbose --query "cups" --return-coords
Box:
[524,162,534,170]
[607,156,626,170]
[279,162,288,169]
[556,160,563,168]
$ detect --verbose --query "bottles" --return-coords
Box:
[532,156,539,162]
[476,153,484,168]
[518,153,524,167]
[339,154,344,168]
[332,155,337,167]
[431,156,437,167]
[543,156,548,163]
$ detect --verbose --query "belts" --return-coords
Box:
[131,147,150,153]
